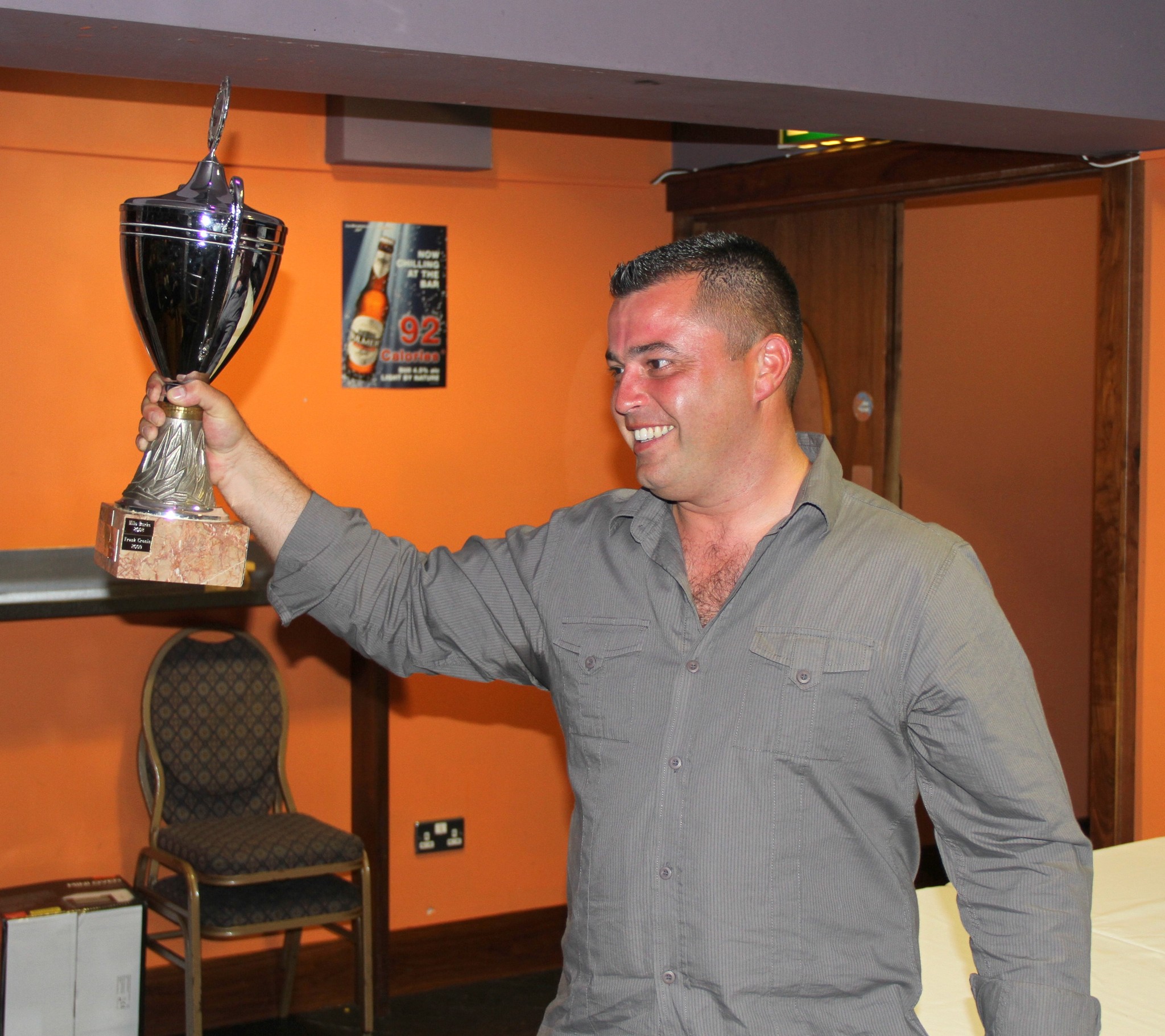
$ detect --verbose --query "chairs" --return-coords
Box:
[134,622,376,1036]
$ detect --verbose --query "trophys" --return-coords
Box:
[92,77,290,588]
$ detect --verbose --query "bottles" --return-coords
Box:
[341,237,396,382]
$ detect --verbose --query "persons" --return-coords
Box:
[135,230,1101,1036]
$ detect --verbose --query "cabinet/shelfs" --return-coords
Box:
[0,876,142,1036]
